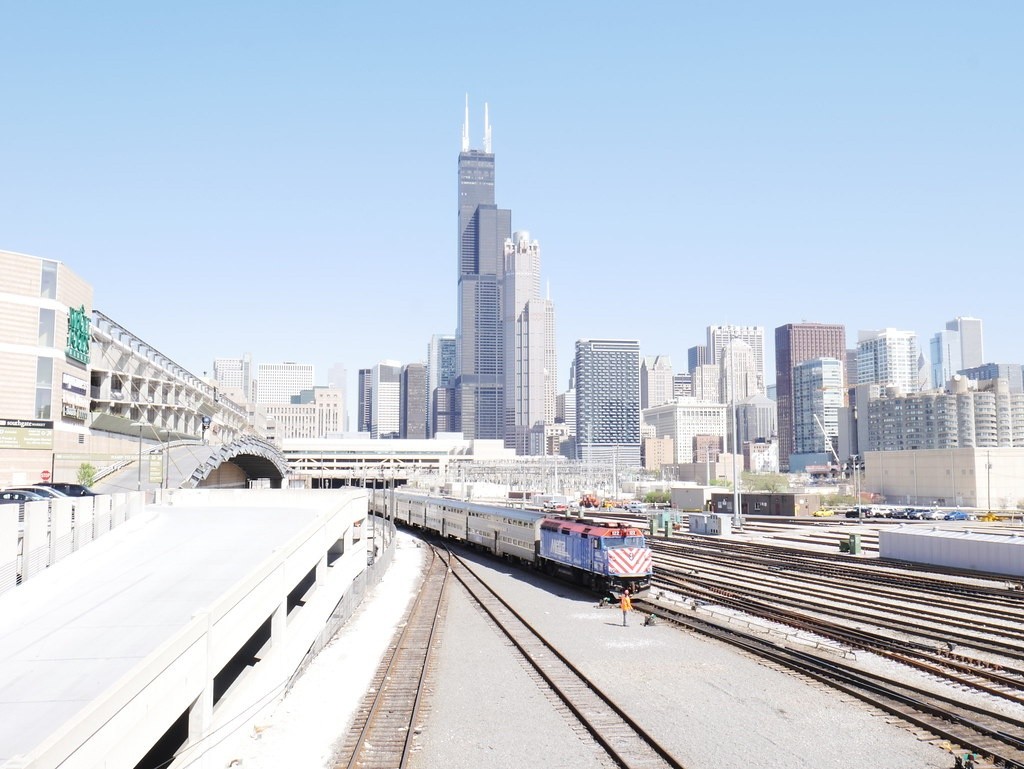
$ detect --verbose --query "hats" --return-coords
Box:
[625,590,629,594]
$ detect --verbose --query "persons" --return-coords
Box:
[619,590,634,627]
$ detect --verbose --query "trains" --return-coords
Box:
[368,486,655,602]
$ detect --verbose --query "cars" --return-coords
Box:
[4,485,69,499]
[813,505,834,517]
[834,504,856,514]
[922,511,947,521]
[944,511,967,521]
[0,490,52,508]
[541,500,561,511]
[33,482,103,497]
[845,504,930,520]
[623,503,645,514]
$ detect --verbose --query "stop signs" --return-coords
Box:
[41,470,51,482]
